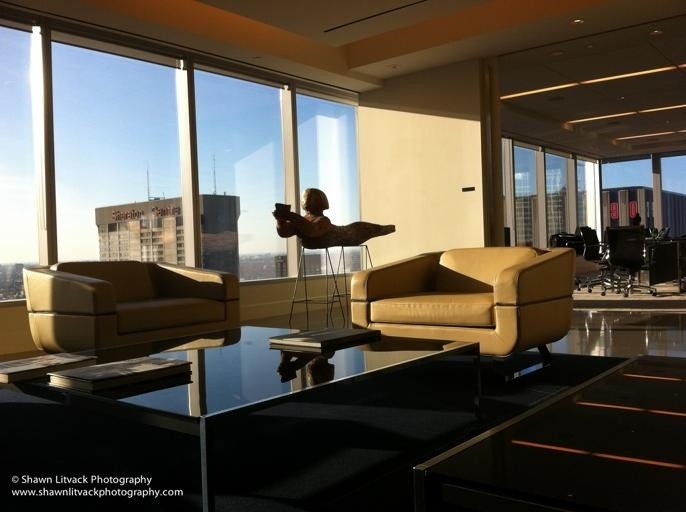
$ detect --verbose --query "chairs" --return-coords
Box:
[22,259,241,352]
[348,244,578,381]
[546,225,659,298]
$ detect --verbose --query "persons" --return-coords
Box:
[271,188,396,249]
[277,347,335,387]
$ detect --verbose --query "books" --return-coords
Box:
[268,328,383,349]
[268,334,380,356]
[44,355,193,395]
[87,370,194,401]
